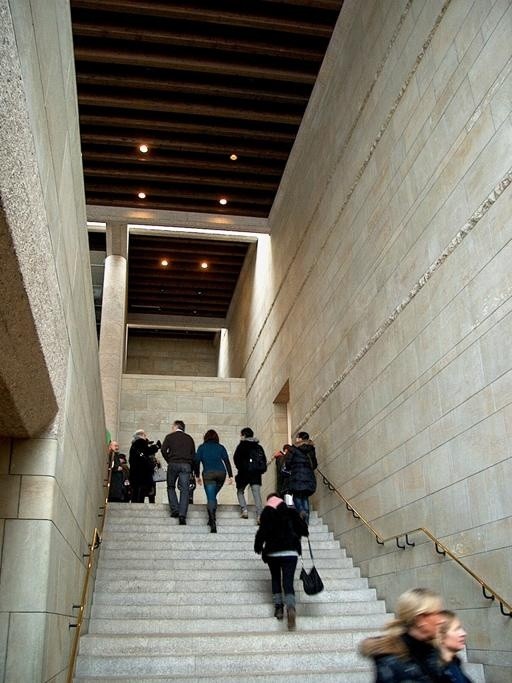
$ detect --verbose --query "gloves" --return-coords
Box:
[156,440,161,449]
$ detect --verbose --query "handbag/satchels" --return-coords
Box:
[300,566,323,594]
[152,463,167,481]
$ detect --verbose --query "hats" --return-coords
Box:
[133,429,144,436]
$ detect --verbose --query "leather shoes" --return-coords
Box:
[274,603,284,618]
[287,605,296,628]
[179,516,186,524]
[170,511,179,517]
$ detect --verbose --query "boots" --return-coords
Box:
[209,509,216,532]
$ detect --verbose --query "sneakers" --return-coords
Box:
[299,510,307,520]
[240,509,248,518]
[256,514,261,525]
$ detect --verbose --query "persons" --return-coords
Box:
[430,609,474,682]
[276,443,291,499]
[194,429,234,532]
[161,419,195,524]
[104,428,163,502]
[283,431,317,525]
[233,426,267,524]
[357,586,455,682]
[252,489,311,631]
[177,472,196,503]
